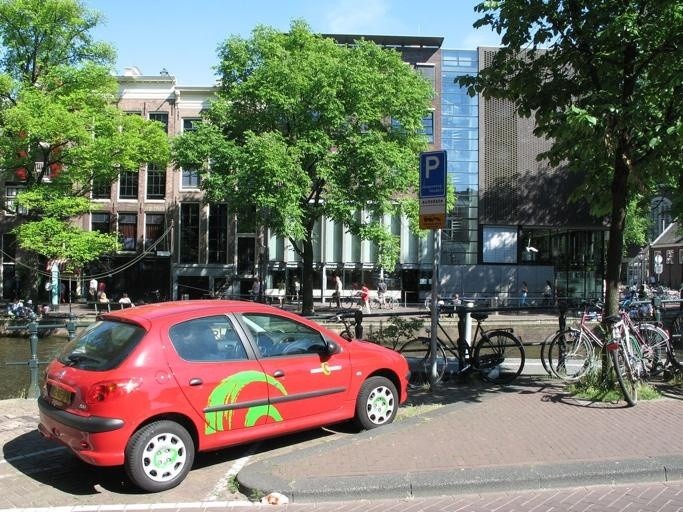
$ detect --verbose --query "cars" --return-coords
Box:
[34,296,411,493]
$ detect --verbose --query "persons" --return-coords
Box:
[519,280,530,311]
[429,295,444,315]
[621,279,683,311]
[4,275,167,319]
[446,293,462,317]
[539,279,554,314]
[251,274,389,314]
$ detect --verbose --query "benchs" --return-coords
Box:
[87,302,131,316]
[435,305,477,317]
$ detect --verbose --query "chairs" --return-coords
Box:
[200,326,220,359]
